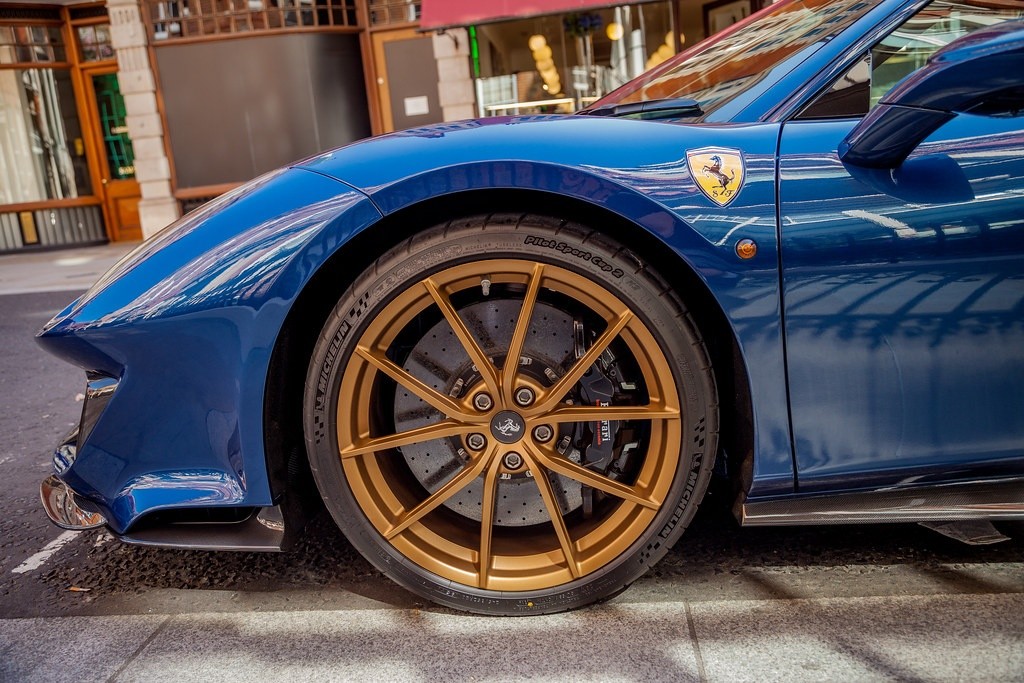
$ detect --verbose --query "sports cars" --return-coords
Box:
[36,2,1022,611]
